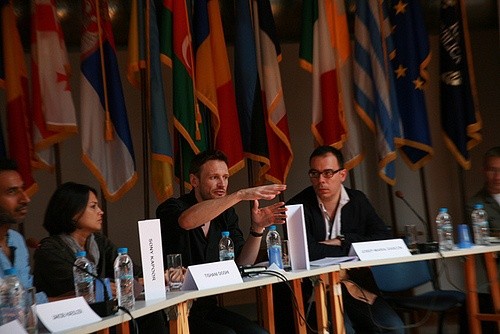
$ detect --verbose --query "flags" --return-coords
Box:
[0,1,482,204]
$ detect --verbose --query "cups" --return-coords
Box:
[166,254,183,290]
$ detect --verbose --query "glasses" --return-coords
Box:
[308,168,343,178]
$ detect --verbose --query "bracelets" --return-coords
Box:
[336,234,346,247]
[249,225,267,237]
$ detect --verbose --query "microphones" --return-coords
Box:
[395,190,439,249]
[53,253,118,317]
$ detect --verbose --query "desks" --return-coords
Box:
[53,240,500,334]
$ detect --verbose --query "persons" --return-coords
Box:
[0,156,48,334]
[280,144,407,334]
[155,147,289,334]
[463,148,500,291]
[32,181,144,297]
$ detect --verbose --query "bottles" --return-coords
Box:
[265,225,283,270]
[0,269,25,330]
[73,251,95,304]
[435,208,454,251]
[114,248,136,310]
[471,204,489,245]
[219,232,235,261]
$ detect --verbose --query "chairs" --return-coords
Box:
[370,261,466,334]
